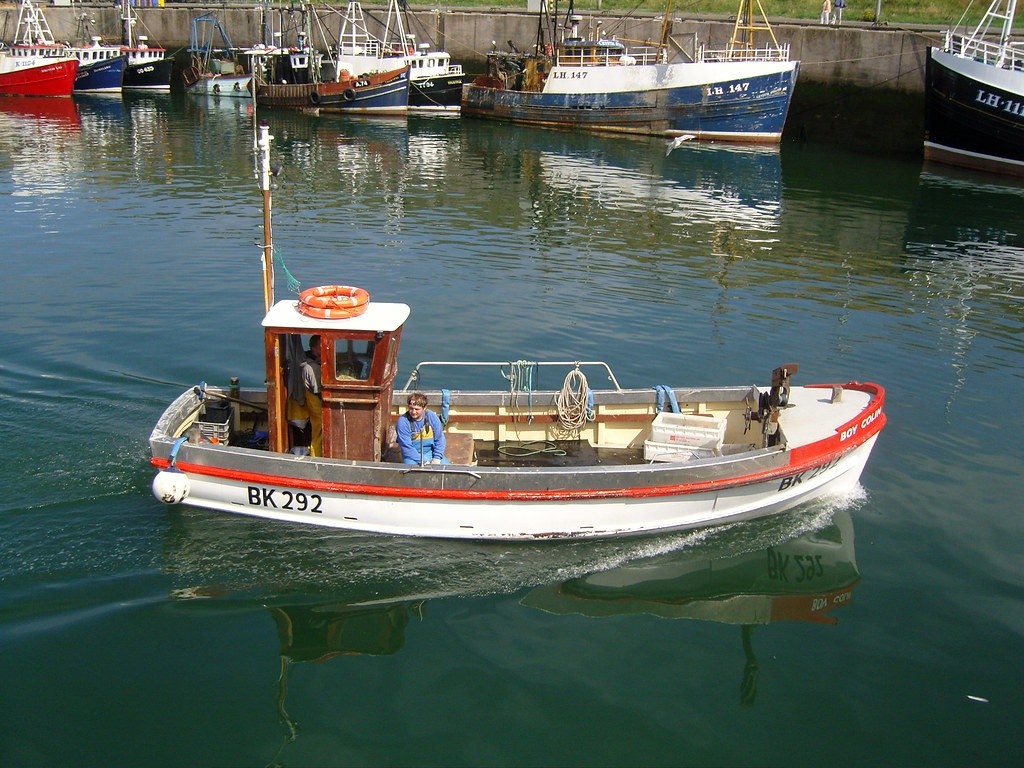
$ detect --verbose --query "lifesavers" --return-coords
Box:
[309,91,321,104]
[343,87,356,102]
[296,284,371,321]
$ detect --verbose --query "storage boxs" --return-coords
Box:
[204,399,231,423]
[644,433,757,462]
[192,406,234,446]
[651,412,728,450]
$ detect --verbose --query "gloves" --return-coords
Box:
[431,458,441,464]
[424,461,430,464]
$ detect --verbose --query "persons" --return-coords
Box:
[299,334,323,457]
[830,0,844,26]
[394,392,452,466]
[819,0,832,25]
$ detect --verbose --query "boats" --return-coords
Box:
[462,0,801,144]
[0,0,466,112]
[924,0,1024,179]
[149,55,887,541]
[172,507,862,741]
